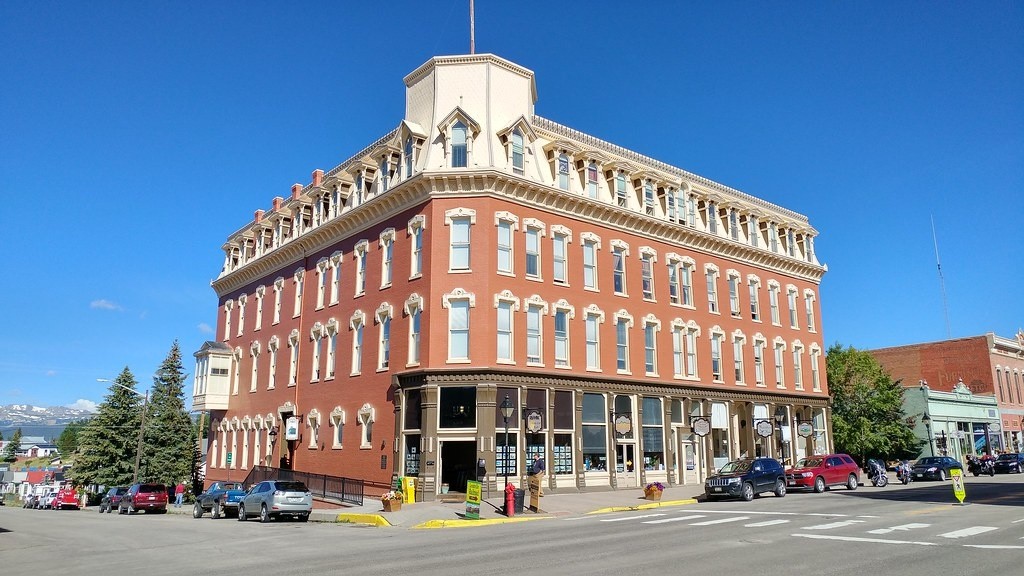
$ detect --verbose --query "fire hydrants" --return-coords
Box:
[504,482,516,517]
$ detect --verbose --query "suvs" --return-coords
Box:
[98,485,130,514]
[237,479,314,523]
[704,455,788,502]
[785,453,861,494]
[118,483,168,515]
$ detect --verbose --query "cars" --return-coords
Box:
[23,489,79,510]
[0,492,4,505]
[994,452,1024,474]
[911,456,963,482]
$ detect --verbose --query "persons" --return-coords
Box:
[534,453,544,496]
[174,482,183,508]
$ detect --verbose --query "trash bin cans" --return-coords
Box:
[504,488,525,514]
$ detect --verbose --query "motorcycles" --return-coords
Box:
[895,459,915,485]
[862,458,889,487]
[966,454,999,477]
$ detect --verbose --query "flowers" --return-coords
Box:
[643,481,665,495]
[380,490,403,506]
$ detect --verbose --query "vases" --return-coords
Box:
[383,499,402,513]
[646,490,662,501]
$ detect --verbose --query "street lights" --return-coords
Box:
[773,406,785,469]
[498,391,515,515]
[920,412,934,456]
[96,379,148,485]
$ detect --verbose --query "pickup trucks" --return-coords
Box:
[193,481,248,520]
